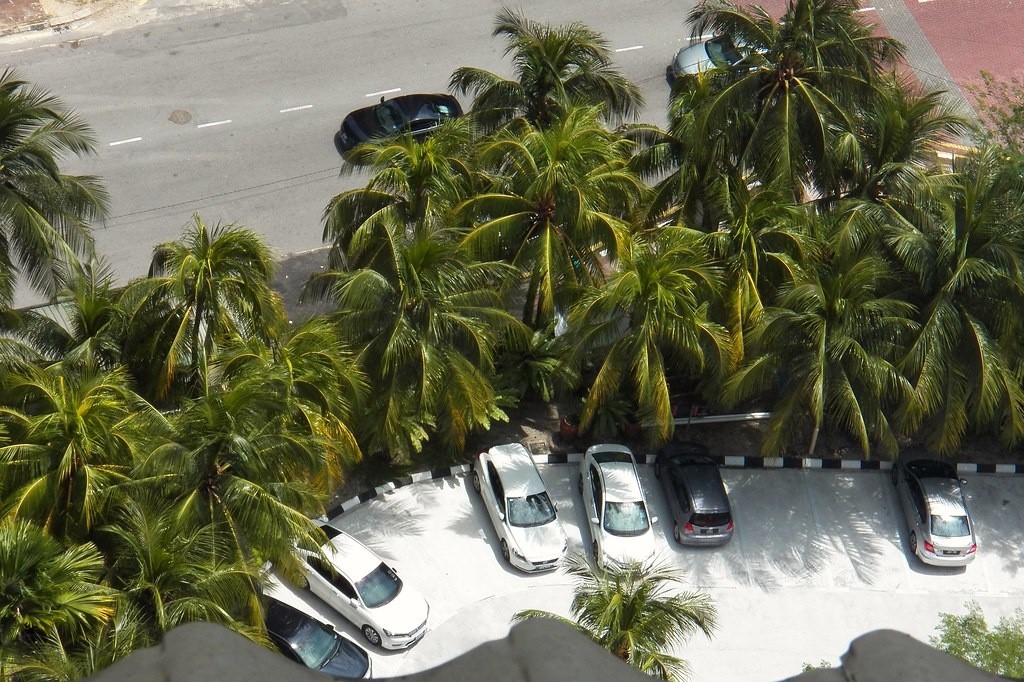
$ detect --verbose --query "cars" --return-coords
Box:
[276,518,430,651]
[892,446,977,567]
[221,594,372,679]
[578,443,658,577]
[473,442,569,574]
[655,441,733,547]
[669,19,793,82]
[339,93,465,163]
